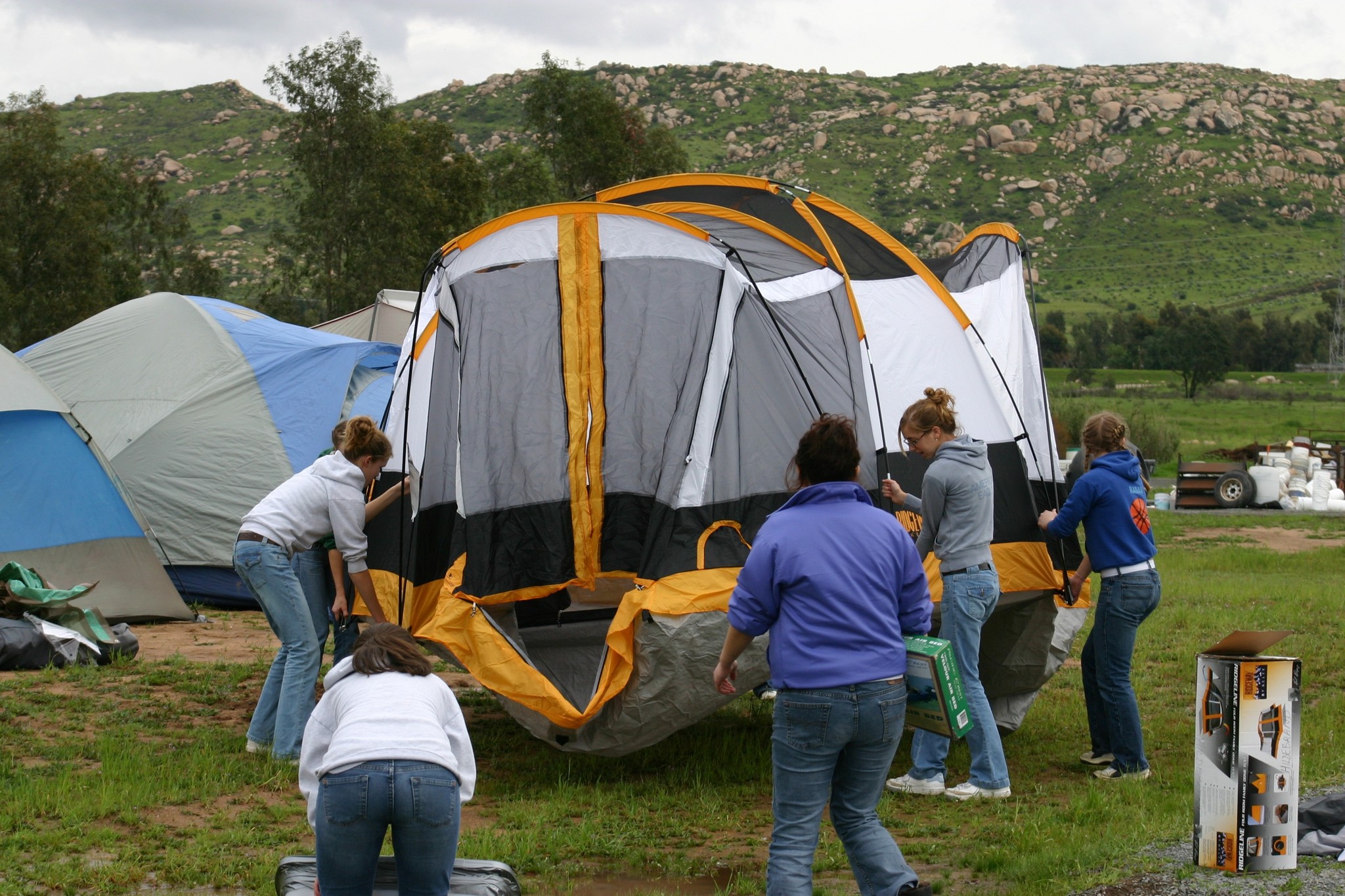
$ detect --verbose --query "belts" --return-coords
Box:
[941,563,991,577]
[1101,559,1155,578]
[237,533,281,546]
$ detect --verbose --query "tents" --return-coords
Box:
[342,171,1089,757]
[0,344,207,623]
[307,289,425,346]
[13,292,403,611]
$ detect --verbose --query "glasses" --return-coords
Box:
[904,430,931,446]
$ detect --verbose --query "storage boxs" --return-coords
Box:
[902,634,974,739]
[1194,630,1302,874]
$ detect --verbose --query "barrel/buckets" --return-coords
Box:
[1274,436,1345,512]
[1154,493,1170,510]
[1248,466,1279,505]
[1066,447,1081,460]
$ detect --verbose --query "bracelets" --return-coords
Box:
[1075,572,1085,580]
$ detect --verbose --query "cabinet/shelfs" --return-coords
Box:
[1267,445,1340,488]
[1175,453,1247,510]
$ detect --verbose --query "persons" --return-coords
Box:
[882,390,1011,800]
[292,420,359,664]
[713,410,934,896]
[232,416,411,767]
[298,624,476,896]
[1066,436,1150,496]
[1038,412,1161,781]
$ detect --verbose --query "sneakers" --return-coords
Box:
[885,773,945,795]
[270,758,300,766]
[1093,765,1149,781]
[246,738,271,754]
[944,782,1011,801]
[1079,751,1116,765]
